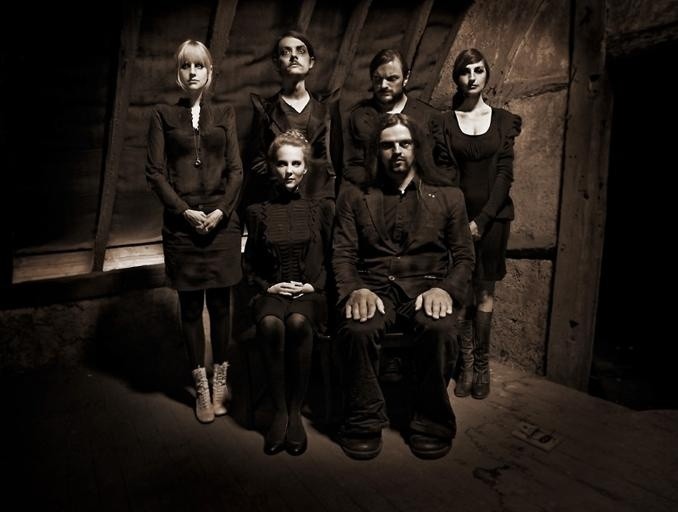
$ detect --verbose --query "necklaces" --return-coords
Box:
[190,127,203,167]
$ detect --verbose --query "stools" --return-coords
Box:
[238,320,336,429]
[380,331,412,417]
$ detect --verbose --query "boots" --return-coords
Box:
[191,365,216,423]
[454,304,494,400]
[211,361,230,416]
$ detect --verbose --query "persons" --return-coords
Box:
[340,48,458,196]
[328,113,476,461]
[241,29,344,228]
[146,40,246,422]
[241,130,335,456]
[442,48,523,397]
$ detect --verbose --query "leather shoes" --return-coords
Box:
[338,430,383,460]
[262,418,309,456]
[408,434,453,459]
